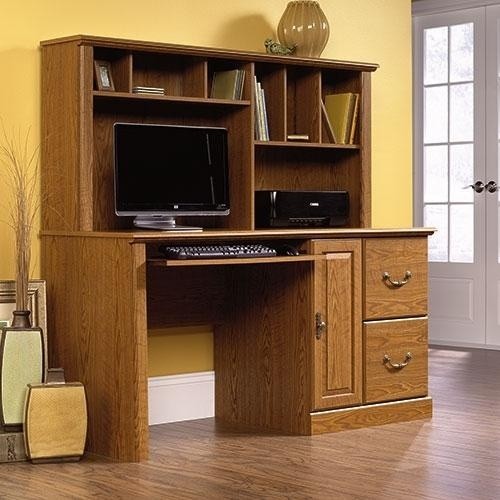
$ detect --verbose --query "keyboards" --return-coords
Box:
[162,242,277,260]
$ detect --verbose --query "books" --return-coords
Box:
[252,75,270,142]
[133,86,165,96]
[320,90,358,146]
[209,69,246,99]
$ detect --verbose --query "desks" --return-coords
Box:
[34,225,435,464]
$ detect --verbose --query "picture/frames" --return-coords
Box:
[92,58,116,93]
[0,278,49,383]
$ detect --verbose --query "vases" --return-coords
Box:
[21,368,90,466]
[0,311,44,431]
[276,1,333,58]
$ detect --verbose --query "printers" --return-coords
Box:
[254,190,351,228]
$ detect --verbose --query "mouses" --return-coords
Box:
[276,244,299,256]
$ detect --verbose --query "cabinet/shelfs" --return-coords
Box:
[38,36,381,232]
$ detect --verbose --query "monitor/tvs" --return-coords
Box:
[111,121,231,230]
